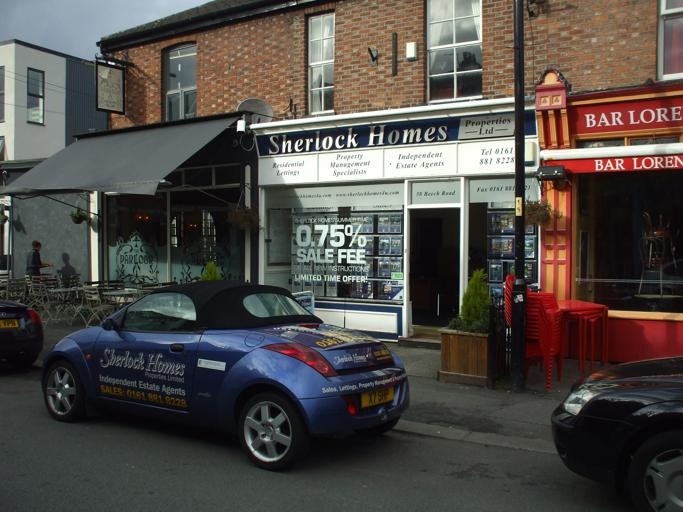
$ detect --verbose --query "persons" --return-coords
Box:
[434,70,468,100]
[18,239,55,310]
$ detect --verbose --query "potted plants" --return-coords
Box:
[0,213,9,227]
[436,268,500,389]
[70,207,89,224]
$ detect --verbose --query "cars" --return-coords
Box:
[550,357,683,512]
[0,297,43,372]
[42,280,410,469]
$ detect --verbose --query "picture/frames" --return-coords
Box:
[486,210,538,303]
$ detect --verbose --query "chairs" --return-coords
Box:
[636,236,676,298]
[0,276,179,328]
[502,275,610,392]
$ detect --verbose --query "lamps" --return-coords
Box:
[535,166,567,184]
[236,110,283,136]
